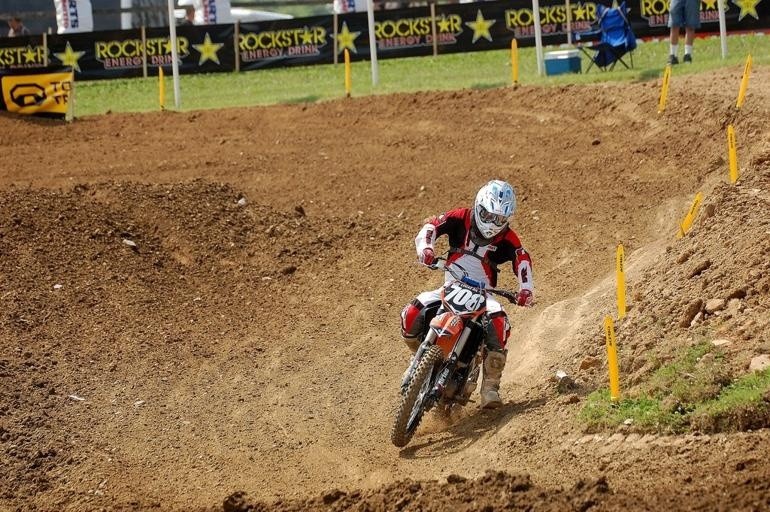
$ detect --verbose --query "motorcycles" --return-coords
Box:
[387,254,522,448]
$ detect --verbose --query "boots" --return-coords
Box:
[481,346,508,409]
[400,328,420,352]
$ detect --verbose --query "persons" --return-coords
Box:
[179,6,196,27]
[5,15,31,38]
[668,0,701,64]
[400,180,533,410]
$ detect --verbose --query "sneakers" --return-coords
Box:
[668,54,691,64]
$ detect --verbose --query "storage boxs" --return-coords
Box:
[543,49,581,75]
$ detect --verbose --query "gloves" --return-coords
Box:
[420,249,434,265]
[517,289,533,306]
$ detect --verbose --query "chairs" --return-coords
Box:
[575,3,638,72]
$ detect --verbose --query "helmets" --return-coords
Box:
[474,180,516,238]
